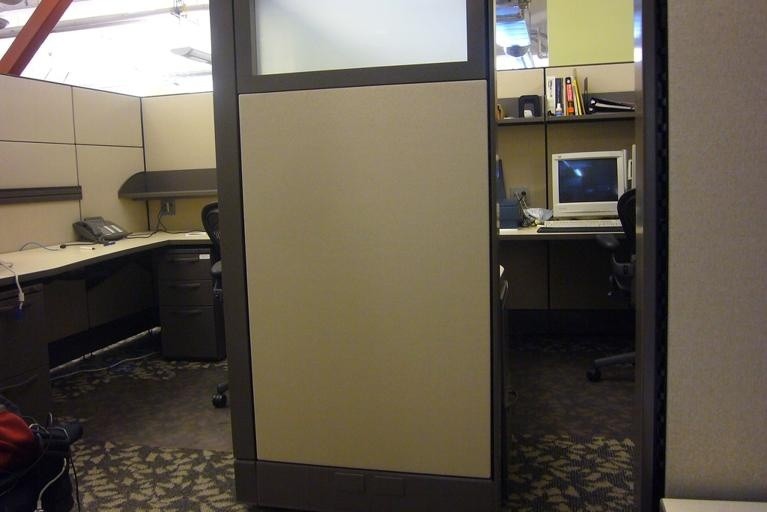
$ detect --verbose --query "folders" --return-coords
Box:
[585,97,634,114]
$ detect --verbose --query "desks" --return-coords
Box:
[497,220,625,243]
[0,230,217,288]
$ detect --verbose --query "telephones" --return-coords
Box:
[73,216,126,241]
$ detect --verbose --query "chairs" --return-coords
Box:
[200,202,230,407]
[585,189,634,382]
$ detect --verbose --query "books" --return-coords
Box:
[544,74,636,116]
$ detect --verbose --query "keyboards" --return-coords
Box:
[546,220,622,227]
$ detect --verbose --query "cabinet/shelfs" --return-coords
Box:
[157,247,226,362]
[0,283,55,427]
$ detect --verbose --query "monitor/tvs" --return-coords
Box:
[552,149,626,217]
